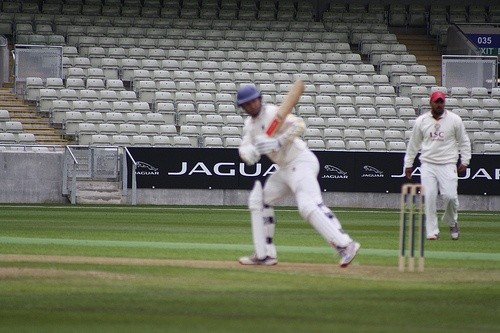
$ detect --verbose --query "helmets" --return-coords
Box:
[235,84,262,108]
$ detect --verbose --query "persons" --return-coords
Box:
[237,85,361,266]
[404,91,471,241]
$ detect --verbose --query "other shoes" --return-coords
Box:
[238,255,278,266]
[426,235,438,240]
[450,223,459,240]
[337,242,361,267]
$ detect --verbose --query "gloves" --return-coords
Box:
[255,136,281,154]
[236,146,261,166]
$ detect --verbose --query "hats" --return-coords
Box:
[430,91,446,102]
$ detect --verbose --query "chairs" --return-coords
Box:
[0,0,500,172]
